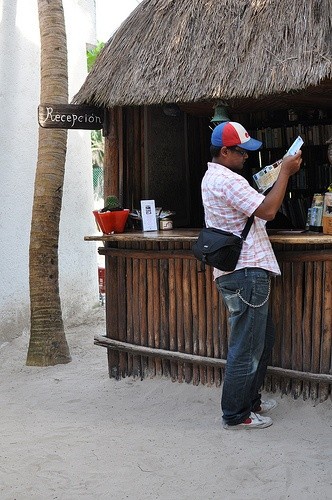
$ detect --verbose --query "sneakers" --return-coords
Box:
[223,411,273,431]
[255,398,278,415]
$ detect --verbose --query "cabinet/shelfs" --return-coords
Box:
[238,107,332,230]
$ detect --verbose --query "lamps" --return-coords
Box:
[210,104,230,123]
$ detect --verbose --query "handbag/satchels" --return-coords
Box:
[192,214,254,272]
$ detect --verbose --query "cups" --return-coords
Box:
[160,218,173,229]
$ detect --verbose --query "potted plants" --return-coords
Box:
[93,195,130,235]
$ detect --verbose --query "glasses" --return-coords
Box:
[227,147,249,155]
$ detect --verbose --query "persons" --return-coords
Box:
[201,120,302,431]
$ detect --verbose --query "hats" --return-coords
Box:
[211,121,262,151]
